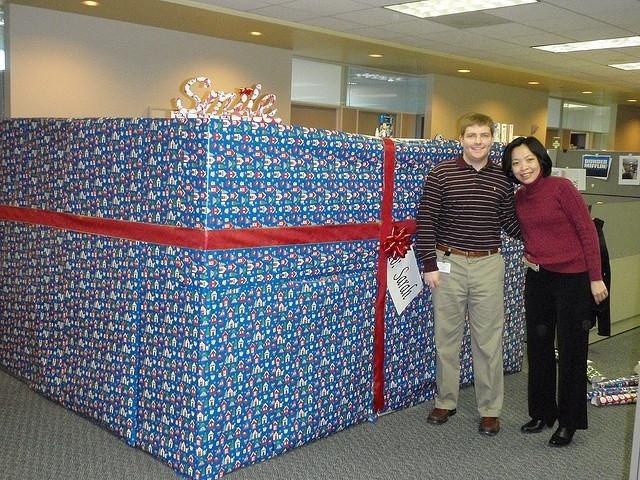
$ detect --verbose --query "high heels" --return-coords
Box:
[522,416,556,434]
[549,424,575,447]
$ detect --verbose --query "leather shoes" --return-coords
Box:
[427,407,457,425]
[479,417,500,436]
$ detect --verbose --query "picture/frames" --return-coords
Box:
[619,156,640,185]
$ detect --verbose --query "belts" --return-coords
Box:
[435,244,498,257]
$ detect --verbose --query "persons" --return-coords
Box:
[502,135,608,446]
[417,114,524,437]
